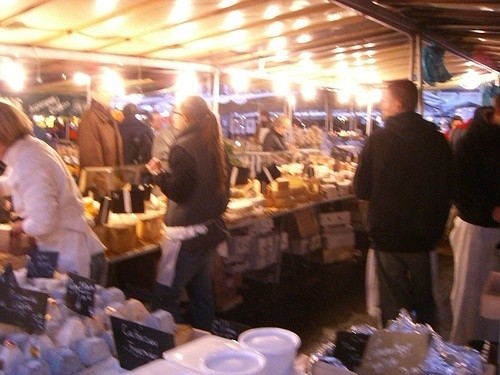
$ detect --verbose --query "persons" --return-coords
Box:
[448,85,500,345]
[151,107,183,201]
[78,77,133,214]
[118,102,162,214]
[444,116,463,142]
[145,95,243,334]
[0,102,109,287]
[254,111,327,173]
[351,78,457,331]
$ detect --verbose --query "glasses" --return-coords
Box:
[170,108,183,118]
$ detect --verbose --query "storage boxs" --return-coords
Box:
[217,206,357,288]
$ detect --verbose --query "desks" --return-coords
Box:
[91,193,362,318]
